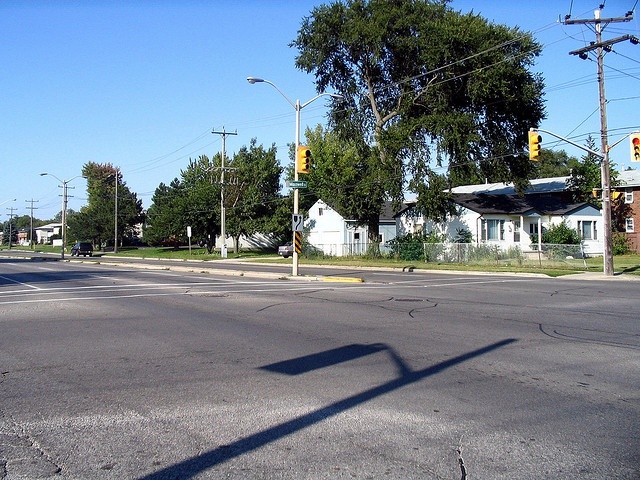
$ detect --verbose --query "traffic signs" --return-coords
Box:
[292,214,303,233]
[186,226,192,255]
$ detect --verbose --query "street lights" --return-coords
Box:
[25,198,39,250]
[5,206,20,249]
[0,198,17,206]
[245,77,345,276]
[39,172,89,259]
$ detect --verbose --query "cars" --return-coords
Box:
[278,242,295,259]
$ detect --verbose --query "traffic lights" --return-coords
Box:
[631,131,640,163]
[296,145,310,174]
[529,126,541,163]
[591,189,599,198]
[613,191,623,201]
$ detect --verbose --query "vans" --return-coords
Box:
[70,241,93,258]
